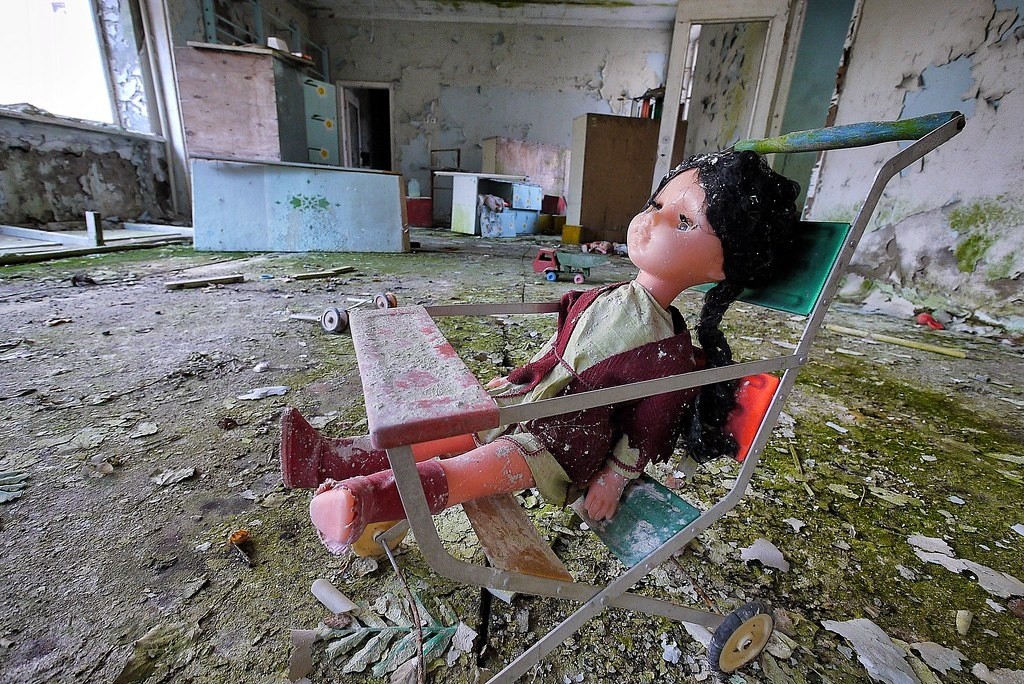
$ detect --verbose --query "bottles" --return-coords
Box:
[408,178,420,197]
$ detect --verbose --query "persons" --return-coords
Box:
[478,193,510,212]
[279,150,803,557]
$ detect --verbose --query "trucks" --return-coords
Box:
[532,248,591,284]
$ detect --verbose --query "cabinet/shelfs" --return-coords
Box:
[452,175,542,235]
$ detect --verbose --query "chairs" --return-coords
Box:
[345,110,970,684]
